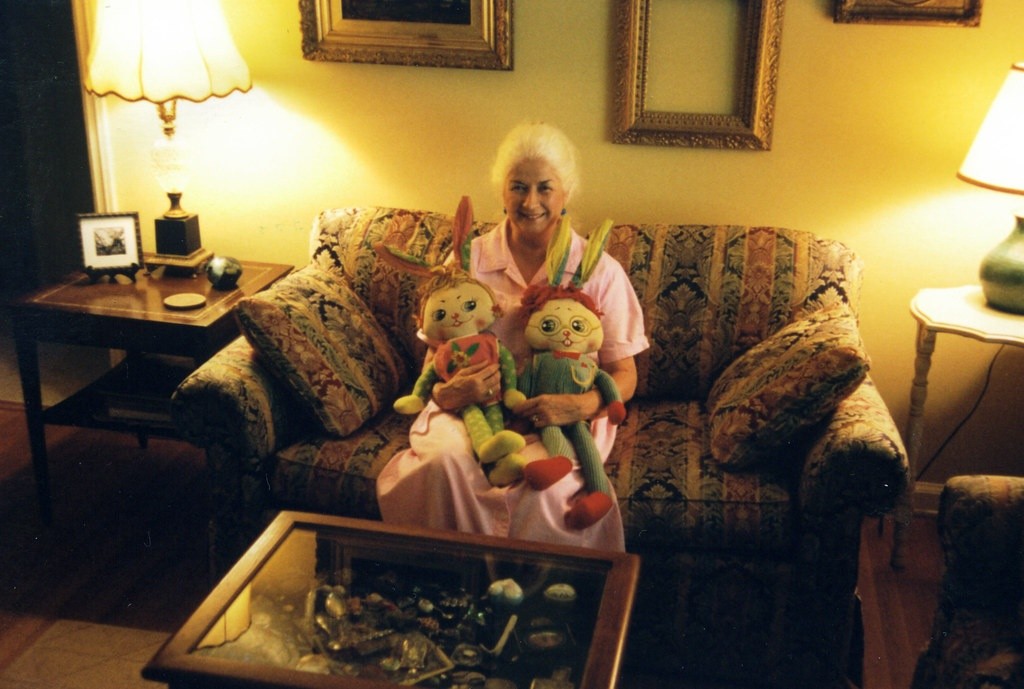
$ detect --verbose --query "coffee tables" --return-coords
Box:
[136,509,642,689]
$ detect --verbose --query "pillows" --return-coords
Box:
[234,262,405,440]
[707,303,869,471]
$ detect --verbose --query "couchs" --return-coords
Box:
[169,208,911,689]
[910,475,1024,689]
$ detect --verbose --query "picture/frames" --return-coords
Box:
[298,0,515,72]
[73,211,145,284]
[613,0,786,152]
[833,0,984,28]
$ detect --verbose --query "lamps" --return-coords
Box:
[955,62,1024,316]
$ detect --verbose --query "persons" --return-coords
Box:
[375,123,648,554]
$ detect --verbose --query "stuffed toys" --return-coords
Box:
[373,197,626,529]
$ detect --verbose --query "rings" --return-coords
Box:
[533,414,538,422]
[488,388,493,396]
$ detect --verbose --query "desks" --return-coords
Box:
[14,251,294,528]
[890,284,1024,568]
